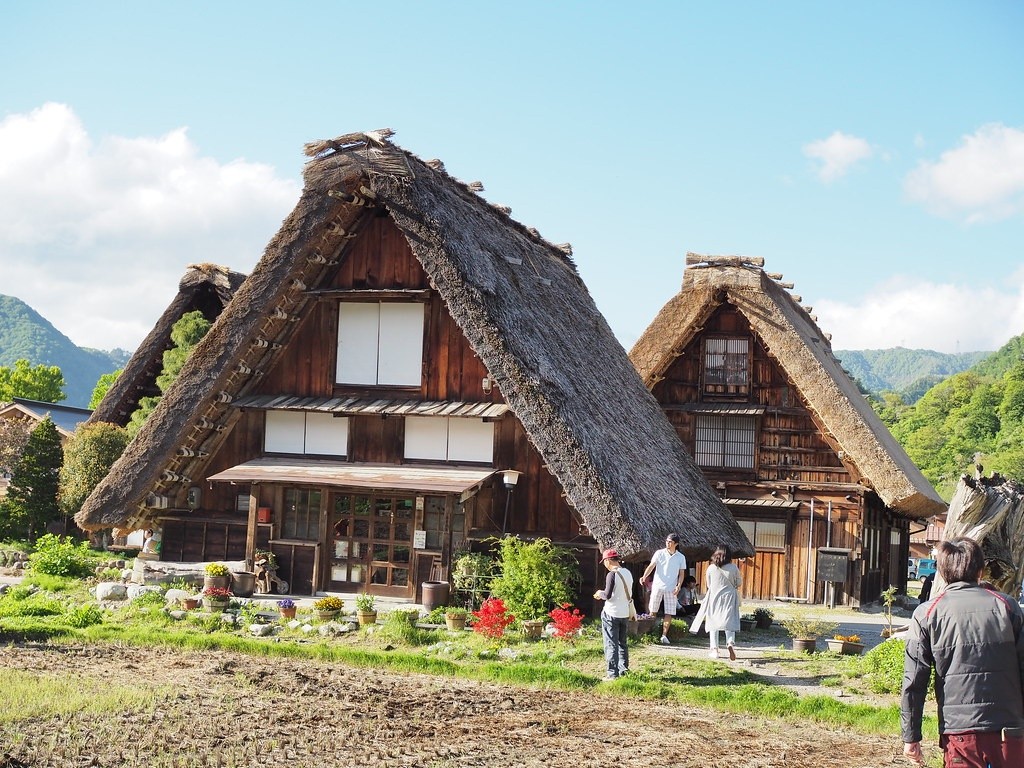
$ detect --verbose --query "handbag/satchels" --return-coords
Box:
[629,599,638,622]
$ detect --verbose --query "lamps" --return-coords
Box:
[495,467,524,490]
[846,494,851,498]
[772,491,776,496]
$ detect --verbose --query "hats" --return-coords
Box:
[599,549,619,563]
[668,534,680,543]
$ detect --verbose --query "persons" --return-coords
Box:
[688,544,741,660]
[900,537,1024,768]
[640,561,686,614]
[678,576,701,614]
[144,529,157,553]
[334,511,349,537]
[639,534,687,643]
[593,550,633,681]
[920,573,935,604]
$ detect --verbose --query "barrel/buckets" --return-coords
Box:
[421,581,451,614]
[231,571,256,598]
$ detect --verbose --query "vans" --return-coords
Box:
[907,557,918,580]
[916,558,937,584]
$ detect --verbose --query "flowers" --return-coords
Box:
[742,607,776,621]
[276,598,295,608]
[313,597,345,611]
[206,563,228,576]
[203,587,229,600]
[833,632,861,643]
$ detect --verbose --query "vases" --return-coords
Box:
[825,639,867,655]
[210,601,229,614]
[233,571,255,597]
[740,619,773,632]
[317,611,341,621]
[278,609,296,619]
[183,599,197,611]
[204,576,230,589]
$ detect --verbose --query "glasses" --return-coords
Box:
[666,540,672,542]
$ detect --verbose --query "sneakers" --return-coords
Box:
[661,635,670,644]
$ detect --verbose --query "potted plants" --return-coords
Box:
[782,616,838,654]
[490,537,569,637]
[356,592,377,625]
[445,606,467,630]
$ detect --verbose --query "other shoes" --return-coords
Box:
[602,673,619,681]
[727,645,736,662]
[620,670,631,677]
[709,651,719,658]
